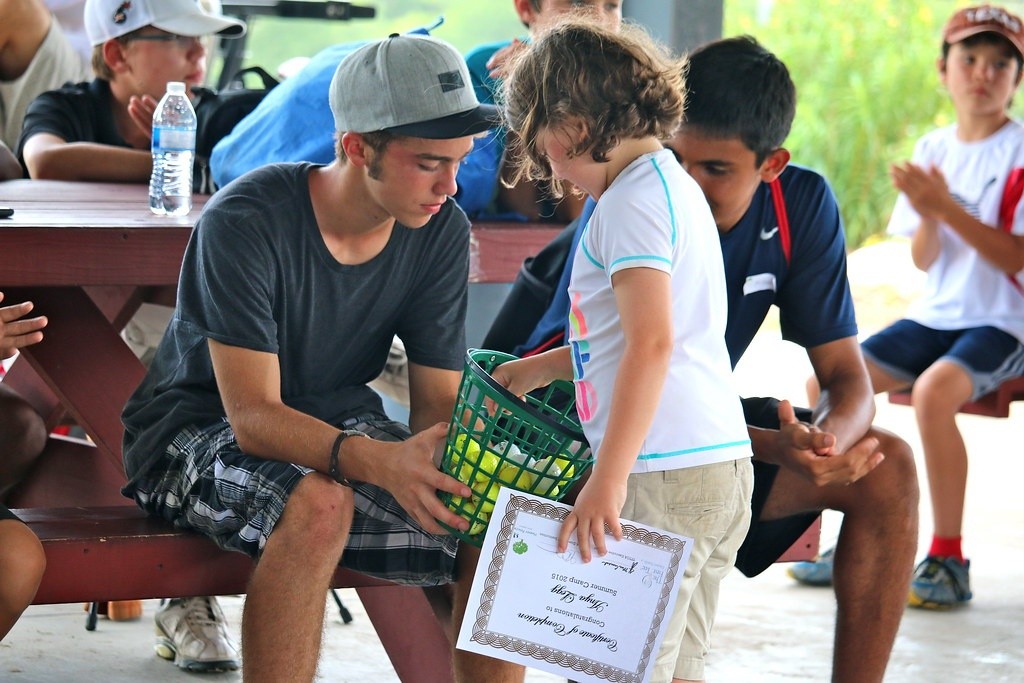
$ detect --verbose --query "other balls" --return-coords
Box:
[440,433,575,535]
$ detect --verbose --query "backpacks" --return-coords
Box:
[185,66,281,150]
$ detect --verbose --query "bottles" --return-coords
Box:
[149,81,198,217]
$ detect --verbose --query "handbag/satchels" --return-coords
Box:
[209,15,509,221]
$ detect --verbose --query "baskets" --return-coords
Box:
[435,347,598,549]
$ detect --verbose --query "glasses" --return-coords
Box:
[117,33,215,51]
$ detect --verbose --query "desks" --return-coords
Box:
[0,174,574,496]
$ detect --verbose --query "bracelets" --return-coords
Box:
[329,426,371,489]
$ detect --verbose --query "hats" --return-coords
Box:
[83,0,250,47]
[941,5,1024,64]
[328,31,509,140]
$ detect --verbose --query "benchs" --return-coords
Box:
[4,508,456,683]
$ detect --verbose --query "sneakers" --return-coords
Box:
[909,552,974,607]
[789,539,840,585]
[154,589,240,673]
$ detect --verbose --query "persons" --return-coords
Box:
[116,28,509,683]
[0,0,249,675]
[784,2,1024,607]
[453,33,921,683]
[460,0,625,222]
